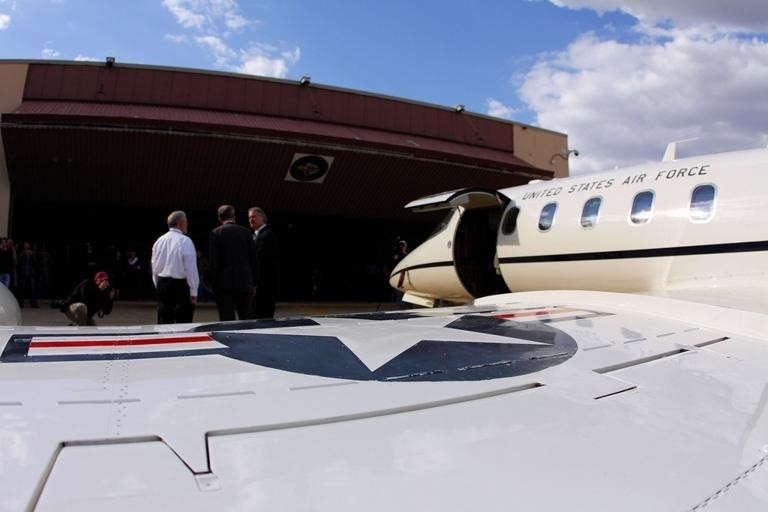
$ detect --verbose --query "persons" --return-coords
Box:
[0,236,154,327]
[203,205,262,320]
[149,210,201,324]
[244,207,282,318]
[393,239,408,261]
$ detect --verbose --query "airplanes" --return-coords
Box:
[0,138,767,511]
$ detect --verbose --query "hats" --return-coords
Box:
[95,272,109,285]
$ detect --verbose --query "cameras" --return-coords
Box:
[104,280,114,293]
